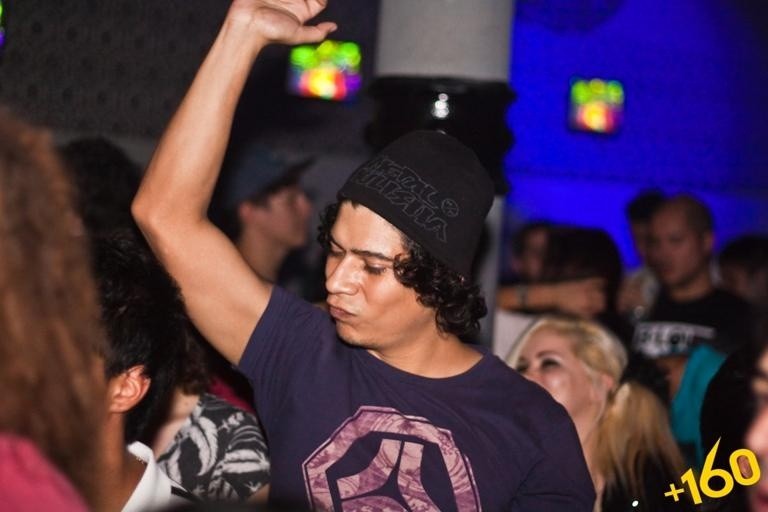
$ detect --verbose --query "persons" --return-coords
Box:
[128,1,605,512]
[2,108,767,512]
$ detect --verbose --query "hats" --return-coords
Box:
[226,154,302,208]
[338,129,495,267]
